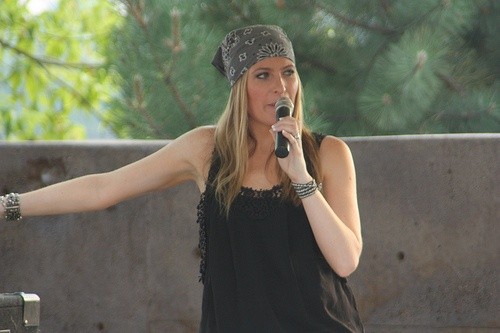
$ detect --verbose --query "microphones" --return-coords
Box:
[274,96,295,158]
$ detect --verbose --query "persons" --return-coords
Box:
[0,24,365,333]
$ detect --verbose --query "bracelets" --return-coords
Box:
[292,179,318,198]
[0,192,22,221]
